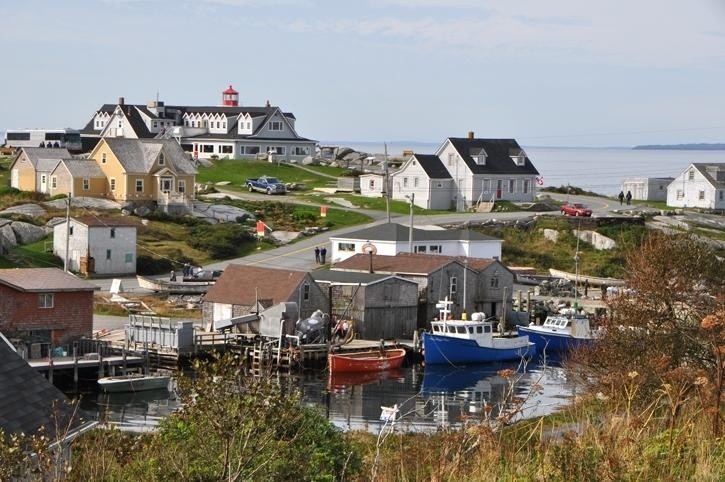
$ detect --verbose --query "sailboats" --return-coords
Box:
[421,257,537,364]
[516,221,602,352]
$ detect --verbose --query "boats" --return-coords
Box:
[328,348,407,374]
[97,373,170,391]
[136,275,216,293]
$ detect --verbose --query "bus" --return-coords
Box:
[5,129,82,151]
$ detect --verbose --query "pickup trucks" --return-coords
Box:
[246,175,287,195]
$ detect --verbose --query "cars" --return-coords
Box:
[560,204,592,217]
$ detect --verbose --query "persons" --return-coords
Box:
[626,191,632,205]
[315,246,327,265]
[462,310,467,320]
[618,191,624,206]
[343,320,348,338]
[379,339,388,355]
[169,261,194,282]
